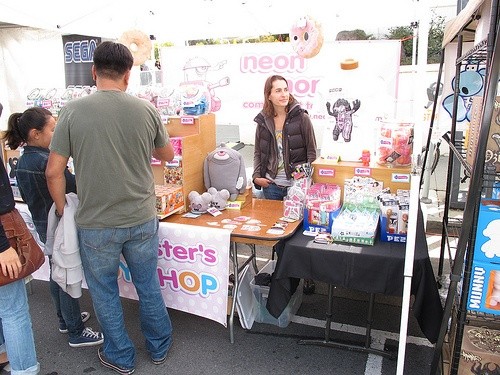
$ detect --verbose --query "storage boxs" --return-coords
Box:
[466,96,500,173]
[448,325,500,375]
[250,259,304,328]
[460,199,500,315]
[303,157,411,245]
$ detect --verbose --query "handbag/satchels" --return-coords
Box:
[0,209,44,287]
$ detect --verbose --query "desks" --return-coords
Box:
[15,196,303,344]
[267,204,448,361]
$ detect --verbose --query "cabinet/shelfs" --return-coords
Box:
[437,0,500,375]
[0,113,216,220]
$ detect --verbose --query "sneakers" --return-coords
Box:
[151,335,174,363]
[59,312,89,333]
[68,328,104,347]
[98,347,135,375]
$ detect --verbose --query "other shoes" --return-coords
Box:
[0,361,11,371]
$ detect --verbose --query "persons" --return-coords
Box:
[0,156,57,375]
[46,41,175,375]
[2,108,104,348]
[252,75,317,296]
